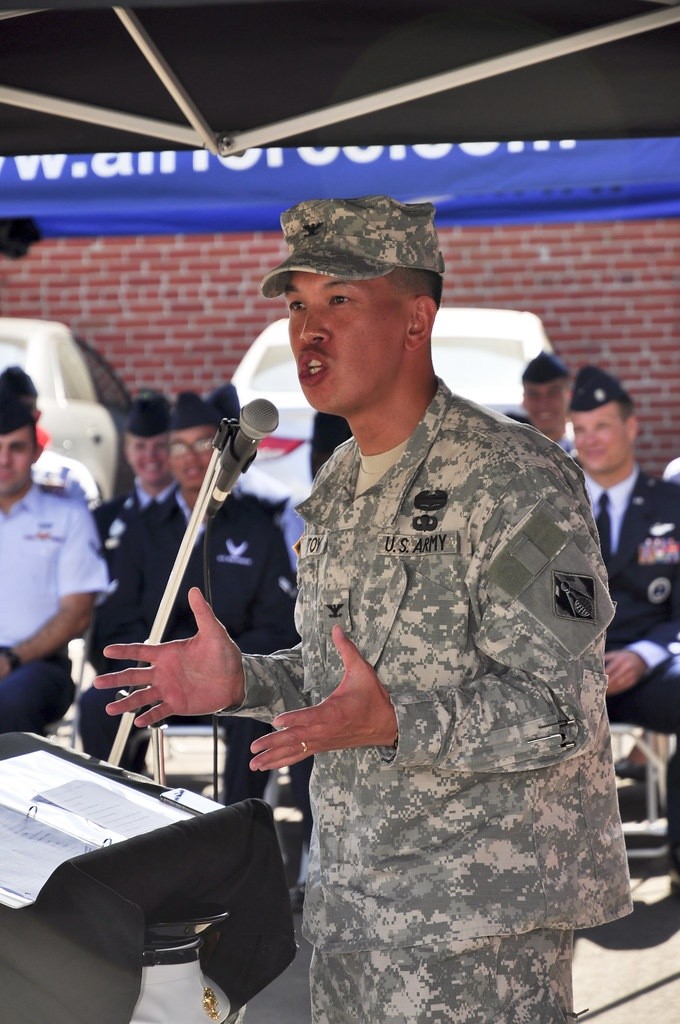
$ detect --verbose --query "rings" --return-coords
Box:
[301,742,308,752]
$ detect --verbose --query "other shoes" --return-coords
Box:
[614,757,649,783]
[288,883,306,916]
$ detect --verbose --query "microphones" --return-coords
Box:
[206,398,280,518]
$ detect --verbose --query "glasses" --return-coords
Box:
[170,437,215,455]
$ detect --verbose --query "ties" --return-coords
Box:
[595,494,610,577]
[142,498,160,517]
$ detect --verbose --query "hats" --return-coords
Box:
[0,366,38,397]
[207,384,240,424]
[168,391,220,430]
[124,388,171,437]
[568,365,620,413]
[0,397,35,434]
[129,902,231,1024]
[259,195,445,299]
[522,350,569,384]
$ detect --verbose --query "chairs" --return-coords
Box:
[34,617,674,822]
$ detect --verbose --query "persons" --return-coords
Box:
[566,363,680,898]
[93,195,634,1024]
[521,350,576,453]
[78,389,302,807]
[91,395,181,583]
[0,366,108,731]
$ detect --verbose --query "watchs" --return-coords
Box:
[0,648,20,672]
